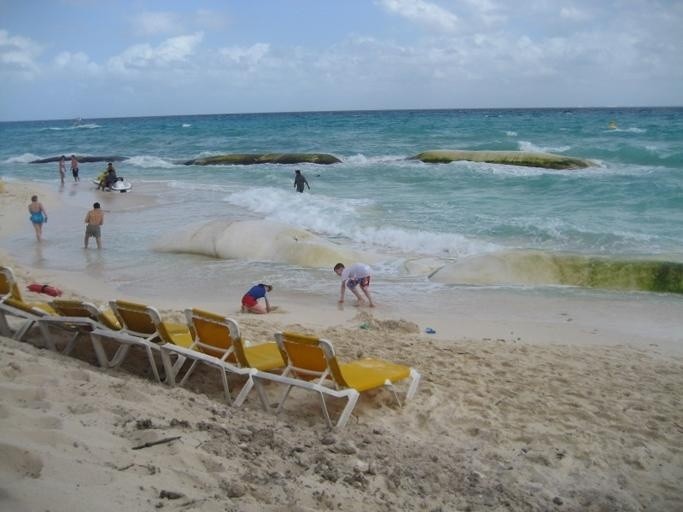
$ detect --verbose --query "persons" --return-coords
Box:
[56,156,69,188]
[334,260,378,308]
[69,156,81,184]
[27,195,49,242]
[240,280,276,316]
[102,163,119,191]
[82,203,104,249]
[292,169,310,193]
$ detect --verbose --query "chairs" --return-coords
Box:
[250,331,421,428]
[160,308,286,408]
[89,300,250,384]
[38,298,169,368]
[0,265,103,341]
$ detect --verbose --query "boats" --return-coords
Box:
[94,177,130,193]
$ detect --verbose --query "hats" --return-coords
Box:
[258,281,272,292]
